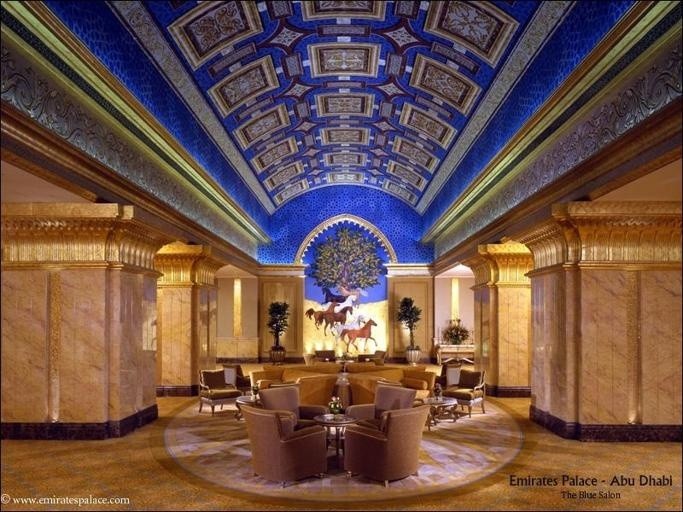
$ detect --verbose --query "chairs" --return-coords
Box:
[199,351,486,488]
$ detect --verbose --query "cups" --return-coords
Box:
[323,413,334,419]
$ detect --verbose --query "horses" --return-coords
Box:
[304,284,377,353]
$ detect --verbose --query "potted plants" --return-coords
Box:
[396,296,422,367]
[442,318,469,345]
[266,301,291,366]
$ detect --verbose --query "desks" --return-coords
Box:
[433,337,476,366]
